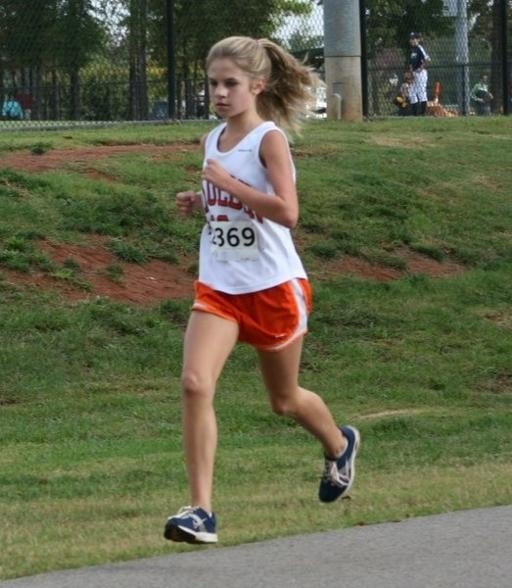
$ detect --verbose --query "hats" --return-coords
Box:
[408,32,420,39]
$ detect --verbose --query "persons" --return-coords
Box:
[390,70,415,116]
[1,88,32,120]
[164,35,361,545]
[407,32,431,116]
[470,74,494,117]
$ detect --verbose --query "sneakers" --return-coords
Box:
[163,507,219,544]
[318,425,360,503]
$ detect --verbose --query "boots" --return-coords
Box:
[407,104,417,116]
[417,102,426,116]
